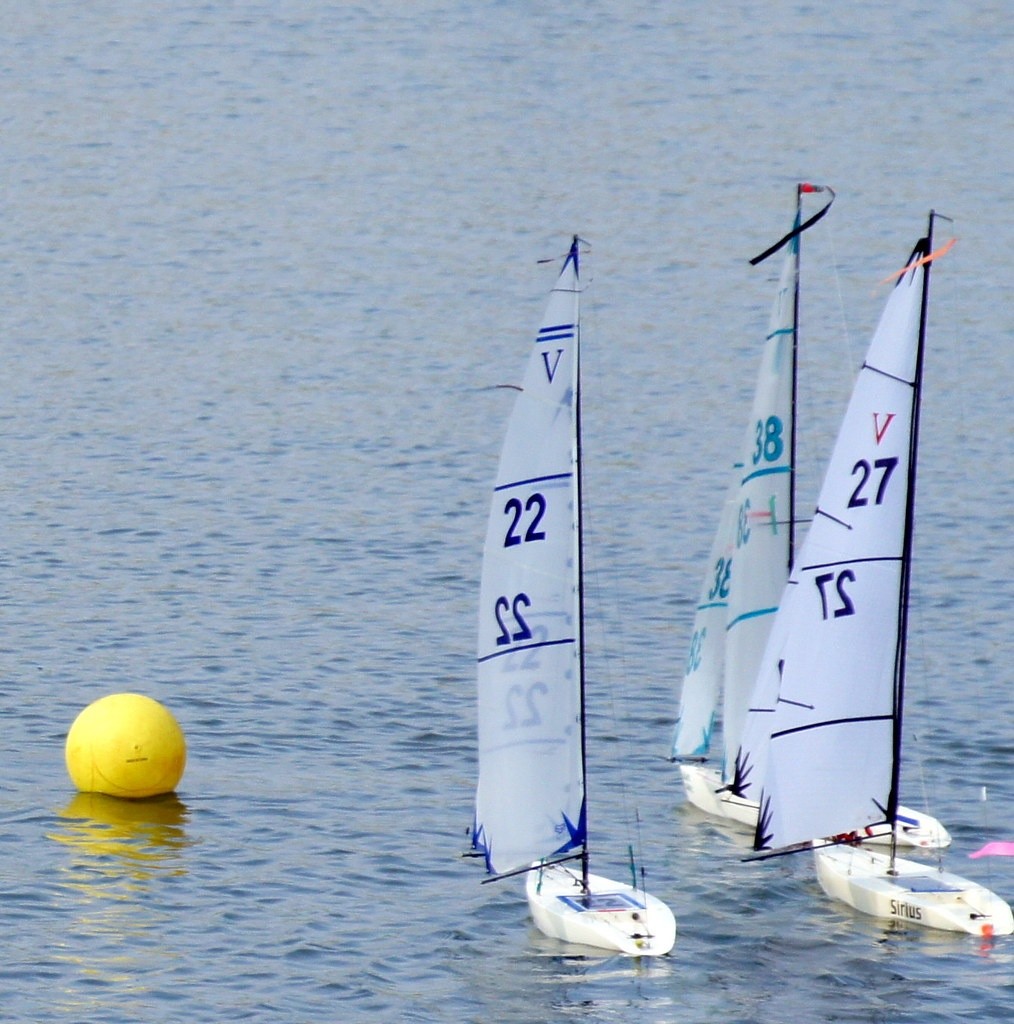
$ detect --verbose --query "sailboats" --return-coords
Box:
[737,210,1013,940]
[461,231,676,955]
[674,181,955,851]
